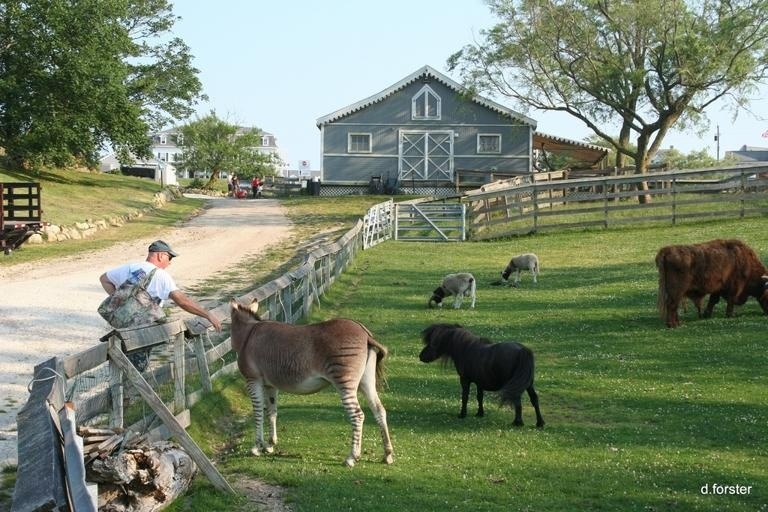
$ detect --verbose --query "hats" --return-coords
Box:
[148,239,179,257]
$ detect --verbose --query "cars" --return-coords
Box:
[238,180,254,197]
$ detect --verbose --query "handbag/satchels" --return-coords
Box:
[97,269,166,328]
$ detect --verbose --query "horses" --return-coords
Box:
[230,297,394,468]
[418,321,546,429]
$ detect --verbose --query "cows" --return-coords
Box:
[501,253,539,283]
[431,272,476,309]
[655,238,768,329]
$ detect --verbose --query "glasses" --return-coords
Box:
[165,253,173,261]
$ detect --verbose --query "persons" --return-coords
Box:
[98,239,221,375]
[227,170,265,199]
